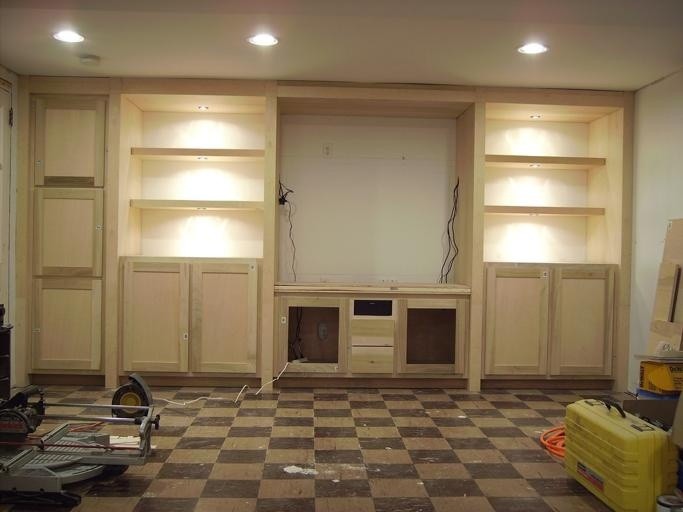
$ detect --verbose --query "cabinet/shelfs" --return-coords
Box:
[0,323,15,403]
[16,74,117,389]
[114,75,275,394]
[276,81,476,391]
[454,84,632,393]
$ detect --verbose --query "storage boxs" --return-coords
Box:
[610,390,680,434]
[637,358,683,397]
[561,396,680,512]
